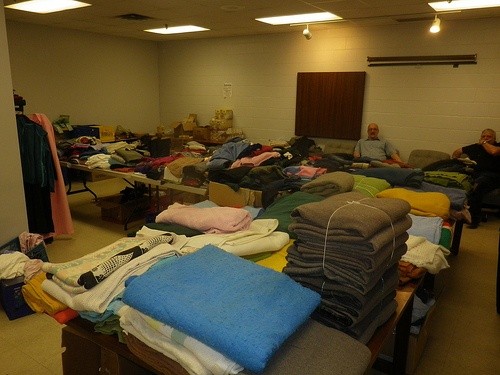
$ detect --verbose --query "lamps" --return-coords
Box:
[302,24,312,40]
[429,14,443,33]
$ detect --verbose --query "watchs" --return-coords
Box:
[481,140,486,145]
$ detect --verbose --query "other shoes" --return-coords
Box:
[467,218,480,228]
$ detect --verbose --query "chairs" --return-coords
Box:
[406,149,449,168]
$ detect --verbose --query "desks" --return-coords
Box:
[99,134,153,145]
[69,202,463,375]
[61,160,215,230]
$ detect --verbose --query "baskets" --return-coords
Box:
[0,236,51,320]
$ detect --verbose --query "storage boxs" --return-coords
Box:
[155,109,244,144]
[207,181,263,210]
[94,191,152,224]
[61,327,145,375]
[0,237,54,320]
[71,123,104,140]
[379,299,439,375]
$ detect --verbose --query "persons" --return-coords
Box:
[353,121,402,165]
[451,128,500,230]
